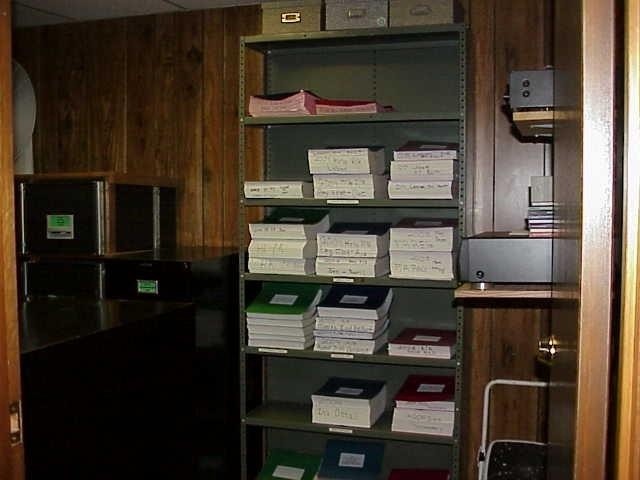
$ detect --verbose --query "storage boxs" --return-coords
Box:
[389,0,454,28]
[261,0,325,34]
[325,0,389,31]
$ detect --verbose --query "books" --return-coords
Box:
[526,176,555,238]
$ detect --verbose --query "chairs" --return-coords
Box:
[476,379,548,480]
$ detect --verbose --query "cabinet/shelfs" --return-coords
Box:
[454,111,554,298]
[239,23,469,480]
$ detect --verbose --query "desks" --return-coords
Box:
[20,245,239,480]
